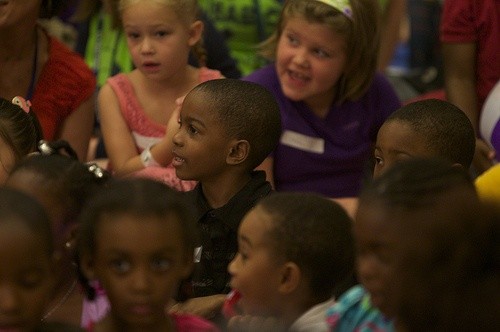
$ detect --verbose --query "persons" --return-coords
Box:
[0,1,500,332]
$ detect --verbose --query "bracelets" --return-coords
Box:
[140,144,163,168]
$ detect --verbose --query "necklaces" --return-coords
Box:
[26,24,39,103]
[40,277,80,325]
